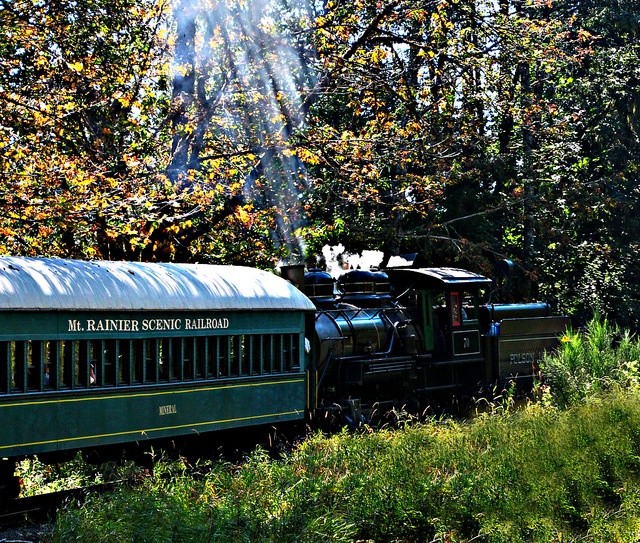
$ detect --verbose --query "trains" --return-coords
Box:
[0,256,570,498]
